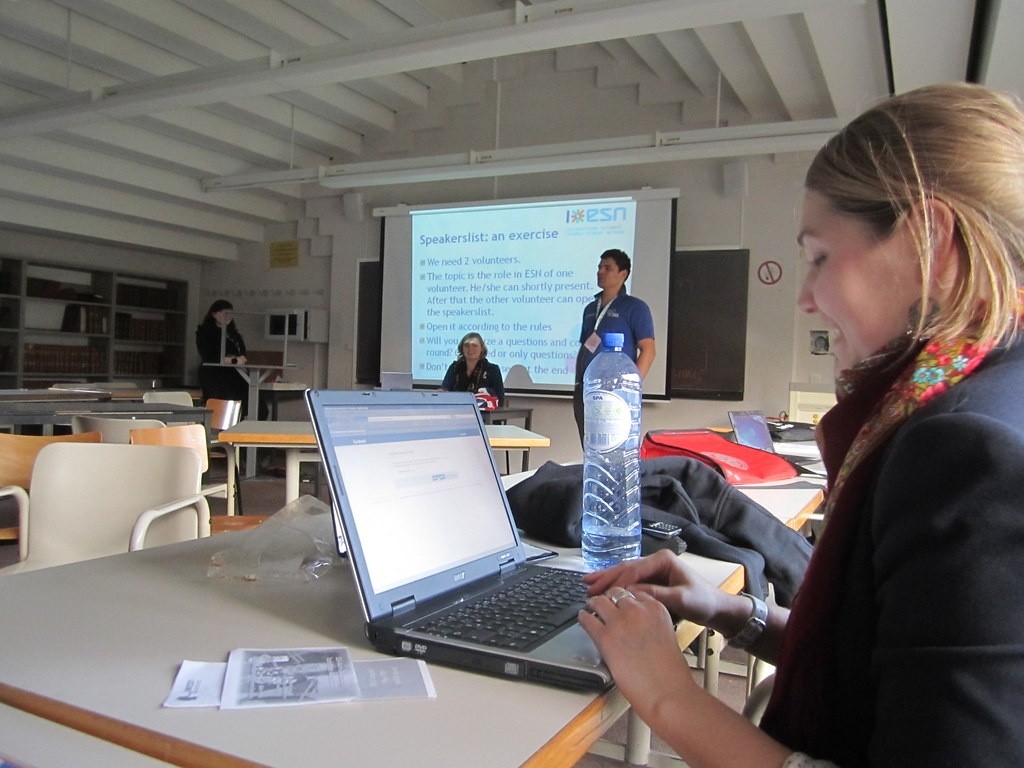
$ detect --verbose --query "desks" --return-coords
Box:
[480,406,534,476]
[0,402,213,435]
[218,419,550,519]
[49,381,204,401]
[205,363,300,477]
[0,461,839,768]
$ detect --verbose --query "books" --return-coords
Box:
[23,283,186,391]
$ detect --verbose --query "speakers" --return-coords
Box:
[342,193,366,223]
[722,161,749,200]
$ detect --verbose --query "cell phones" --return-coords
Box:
[642,519,682,539]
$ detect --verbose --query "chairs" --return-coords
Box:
[0,385,244,581]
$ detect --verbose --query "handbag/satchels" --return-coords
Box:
[640,429,801,484]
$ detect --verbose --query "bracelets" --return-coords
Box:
[722,590,767,650]
[231,355,238,365]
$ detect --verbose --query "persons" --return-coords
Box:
[572,248,658,453]
[438,332,505,425]
[577,80,1024,768]
[196,299,248,424]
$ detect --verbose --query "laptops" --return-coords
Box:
[305,391,684,694]
[728,409,822,466]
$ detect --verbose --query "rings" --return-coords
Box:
[610,591,640,606]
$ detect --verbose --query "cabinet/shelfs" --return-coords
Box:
[0,253,189,390]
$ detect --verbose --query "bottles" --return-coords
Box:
[582,333,644,571]
